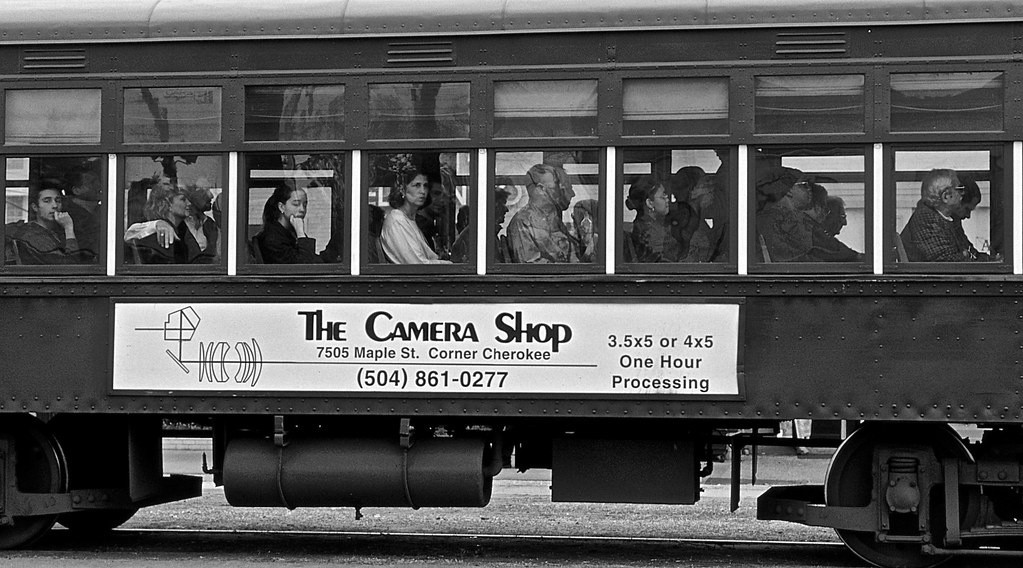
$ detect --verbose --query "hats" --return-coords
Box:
[495,186,512,202]
[755,166,804,201]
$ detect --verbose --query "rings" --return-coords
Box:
[160,234,164,236]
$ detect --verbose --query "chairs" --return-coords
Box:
[759,234,773,264]
[130,238,144,265]
[895,232,909,261]
[626,230,640,263]
[251,236,264,264]
[375,237,386,265]
[500,233,512,264]
[12,238,36,266]
[574,198,598,263]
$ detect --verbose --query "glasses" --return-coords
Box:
[937,184,965,200]
[648,194,668,203]
[815,205,832,215]
[794,180,809,188]
[837,214,847,219]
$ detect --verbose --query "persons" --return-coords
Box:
[17,167,101,263]
[369,169,451,264]
[256,184,327,263]
[507,164,593,264]
[124,174,222,263]
[758,167,866,261]
[450,188,511,262]
[899,169,1003,262]
[628,146,769,262]
[778,419,812,454]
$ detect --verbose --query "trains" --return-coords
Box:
[0,0,1023,568]
